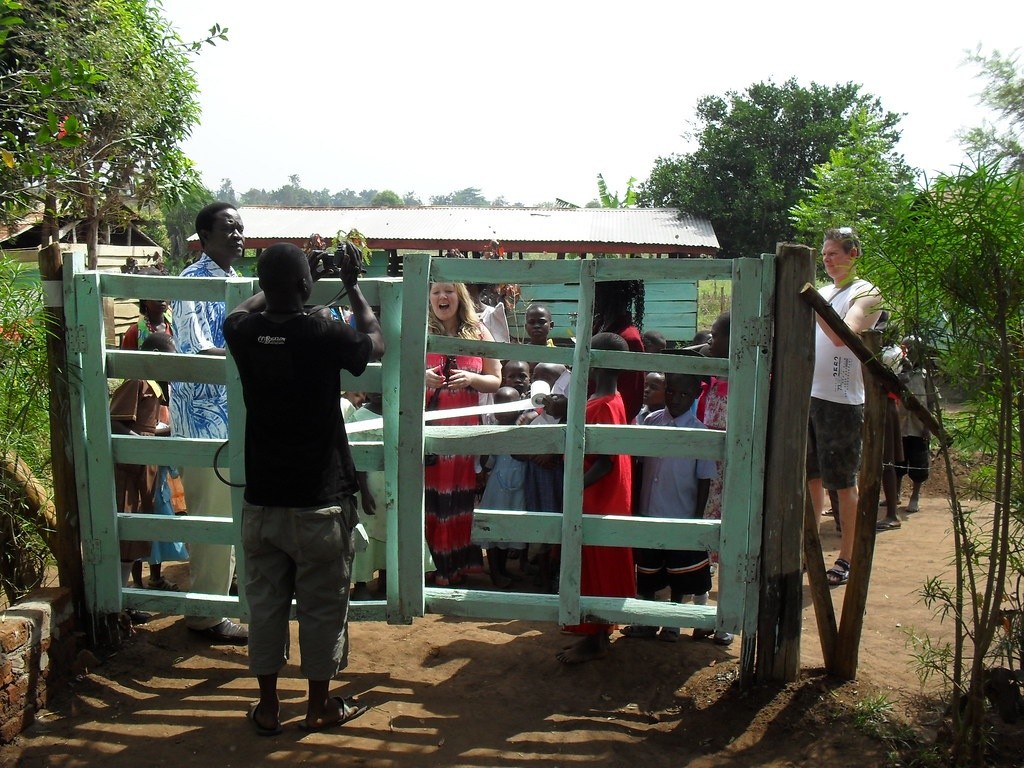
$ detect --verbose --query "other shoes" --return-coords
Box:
[713,622,737,644]
[428,568,451,590]
[693,624,714,637]
[124,606,155,625]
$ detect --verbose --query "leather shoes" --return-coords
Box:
[199,615,253,646]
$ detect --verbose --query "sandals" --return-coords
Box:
[623,618,658,638]
[150,576,182,595]
[825,556,854,585]
[657,622,684,643]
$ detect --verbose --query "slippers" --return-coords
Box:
[874,517,902,532]
[299,691,372,732]
[823,506,833,515]
[243,694,285,736]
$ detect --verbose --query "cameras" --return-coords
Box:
[321,242,346,276]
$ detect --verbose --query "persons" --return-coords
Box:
[110,201,933,665]
[222,240,386,735]
